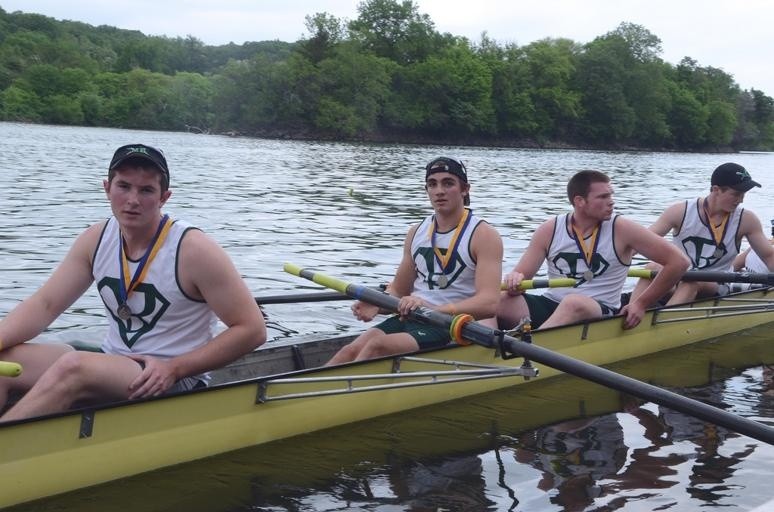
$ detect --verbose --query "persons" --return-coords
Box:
[0,144,267,422]
[729,220,774,292]
[323,157,502,367]
[628,162,774,307]
[495,170,690,329]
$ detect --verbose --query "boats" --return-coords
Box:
[0,279,774,512]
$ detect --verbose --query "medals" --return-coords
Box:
[437,275,449,288]
[583,270,594,281]
[116,304,132,320]
[713,248,724,258]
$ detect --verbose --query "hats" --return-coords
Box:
[424,154,473,208]
[711,161,764,194]
[108,141,171,189]
